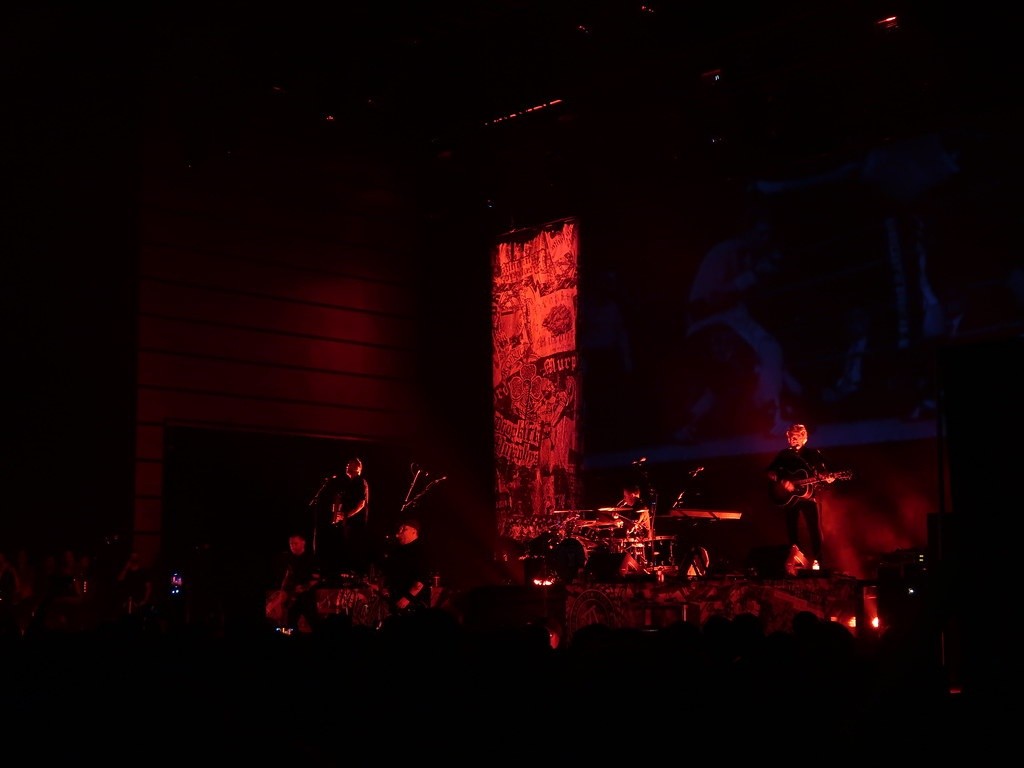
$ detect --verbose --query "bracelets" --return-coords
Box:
[404,592,415,602]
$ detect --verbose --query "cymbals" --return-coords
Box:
[553,509,592,514]
[593,507,633,512]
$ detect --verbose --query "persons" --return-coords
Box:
[0,567,1024,768]
[339,457,369,547]
[381,518,431,615]
[768,423,836,577]
[279,535,326,636]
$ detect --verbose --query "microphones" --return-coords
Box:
[631,456,646,466]
[689,466,705,478]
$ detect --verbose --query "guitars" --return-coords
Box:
[768,466,854,506]
